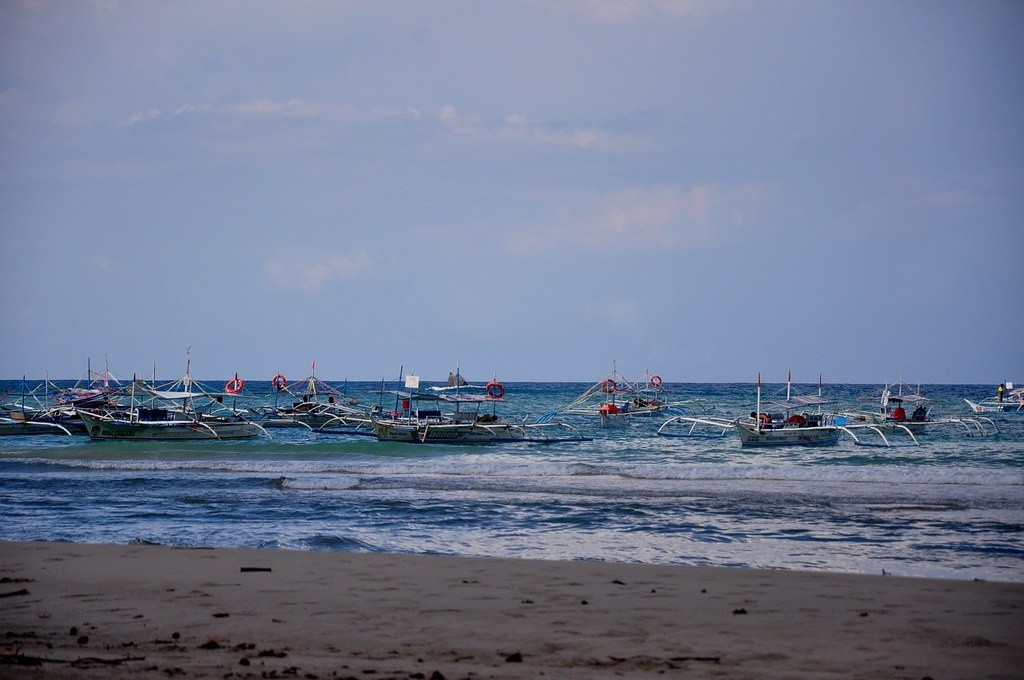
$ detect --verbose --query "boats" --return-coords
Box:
[601,412,627,429]
[869,413,930,435]
[734,421,842,449]
[629,405,670,417]
[265,410,341,427]
[964,387,1024,413]
[76,409,264,440]
[370,416,522,441]
[0,422,81,435]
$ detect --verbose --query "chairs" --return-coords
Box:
[783,423,799,429]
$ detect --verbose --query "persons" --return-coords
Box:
[998,383,1004,404]
[750,411,772,430]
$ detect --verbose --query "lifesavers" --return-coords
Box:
[225,378,244,394]
[487,382,505,397]
[651,376,663,387]
[755,415,769,429]
[273,375,286,389]
[601,380,616,393]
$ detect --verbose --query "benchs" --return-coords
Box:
[419,415,450,426]
[450,411,477,424]
